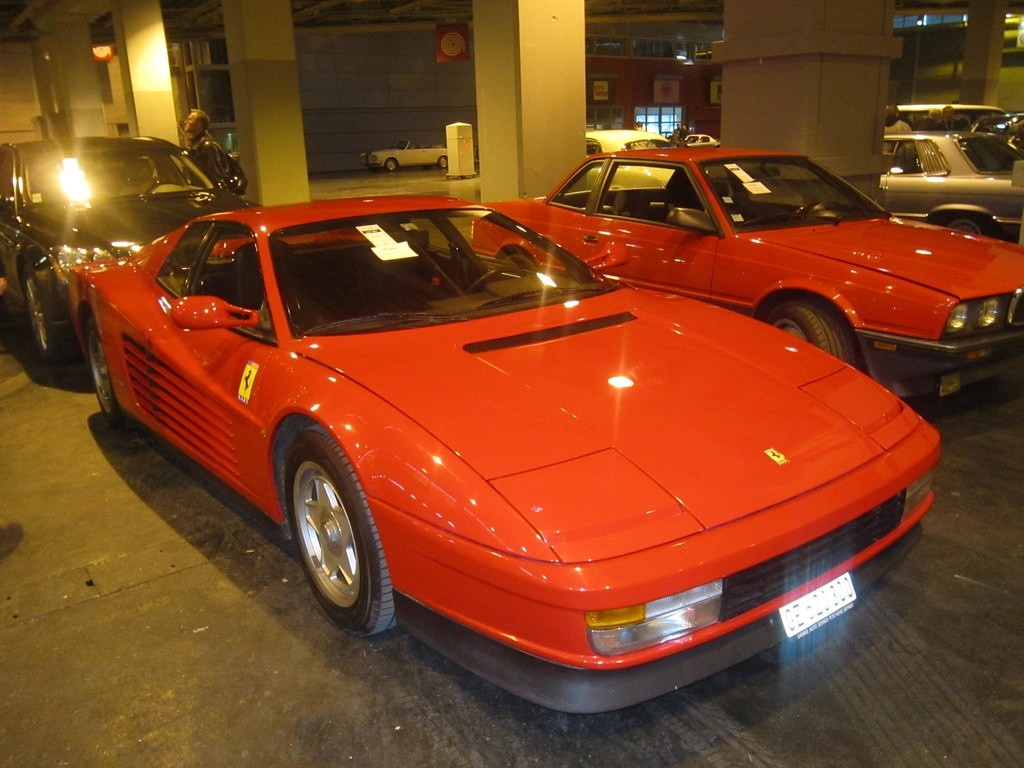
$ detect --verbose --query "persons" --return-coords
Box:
[884,103,962,134]
[633,120,687,140]
[183,108,229,187]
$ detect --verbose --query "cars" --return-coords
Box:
[0,136,259,364]
[880,130,1024,237]
[468,146,1024,400]
[685,134,719,146]
[896,103,1024,150]
[359,138,448,172]
[584,130,675,190]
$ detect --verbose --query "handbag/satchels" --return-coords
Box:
[224,155,248,195]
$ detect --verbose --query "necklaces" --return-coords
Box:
[945,120,954,131]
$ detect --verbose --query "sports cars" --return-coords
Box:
[68,195,942,717]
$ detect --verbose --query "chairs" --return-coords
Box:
[343,230,450,308]
[116,159,156,195]
[234,239,340,332]
[666,170,705,218]
[730,168,794,215]
